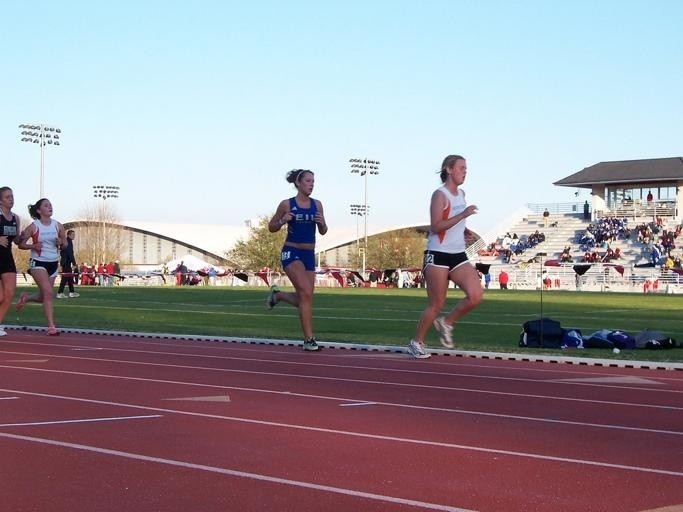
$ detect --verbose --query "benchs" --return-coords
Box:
[465,200,682,287]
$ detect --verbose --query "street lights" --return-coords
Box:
[93,184,121,263]
[18,123,61,200]
[349,158,381,272]
[350,203,370,249]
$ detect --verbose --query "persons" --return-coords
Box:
[542,270,548,290]
[584,200,590,219]
[560,245,574,267]
[621,194,632,202]
[577,215,682,284]
[321,263,426,288]
[0,186,29,336]
[647,190,654,205]
[66,260,120,286]
[15,200,68,338]
[488,230,546,264]
[454,268,491,289]
[552,221,559,228]
[161,262,170,275]
[408,153,484,361]
[268,168,329,355]
[543,208,549,228]
[499,269,509,289]
[174,261,267,286]
[57,230,80,298]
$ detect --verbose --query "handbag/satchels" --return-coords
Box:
[520,318,677,349]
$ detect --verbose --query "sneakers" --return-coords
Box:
[47,326,57,336]
[267,285,280,309]
[408,339,431,359]
[433,317,455,349]
[303,337,321,351]
[56,292,69,299]
[16,292,27,313]
[69,292,80,297]
